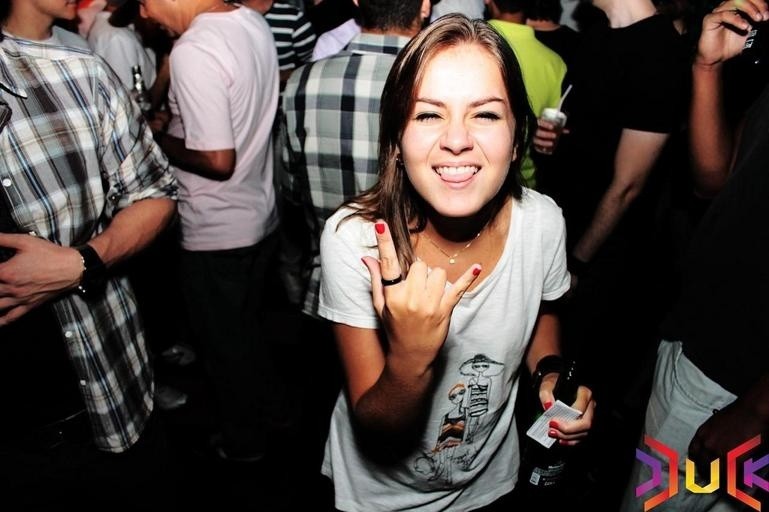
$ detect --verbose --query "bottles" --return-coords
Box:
[520,342,591,491]
[740,14,767,71]
[127,64,151,116]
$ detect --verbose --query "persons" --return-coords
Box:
[0,0,769,512]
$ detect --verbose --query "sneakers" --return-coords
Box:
[152,341,196,367]
[153,384,190,409]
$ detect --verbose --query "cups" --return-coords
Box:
[533,107,568,155]
